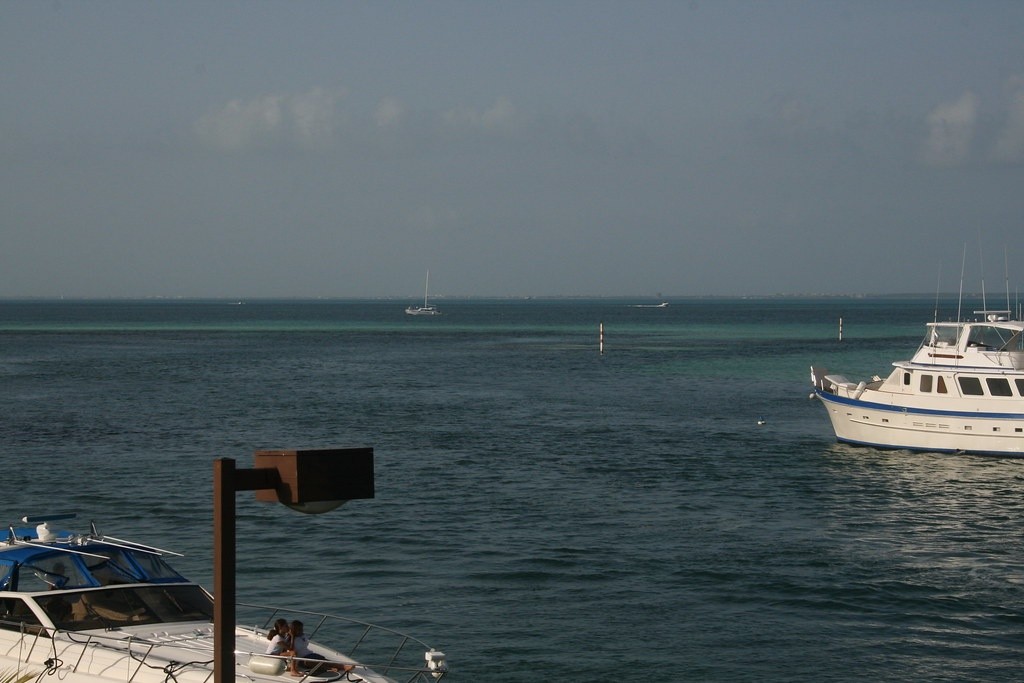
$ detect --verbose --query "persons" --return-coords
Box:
[46,562,69,604]
[286,620,356,673]
[264,618,304,677]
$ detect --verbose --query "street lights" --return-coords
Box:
[211,445,378,683]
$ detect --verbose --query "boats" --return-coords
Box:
[810,242,1024,458]
[0,514,395,682]
